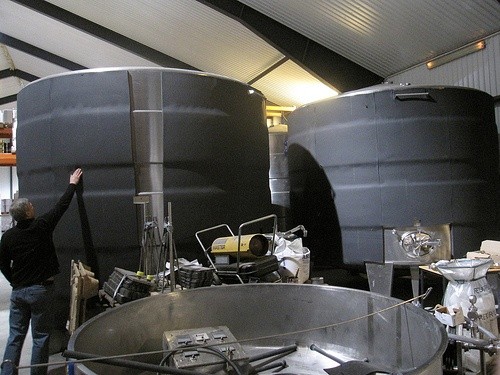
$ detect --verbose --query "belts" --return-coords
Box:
[35,280,54,286]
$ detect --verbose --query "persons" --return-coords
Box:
[0,167,82,375]
